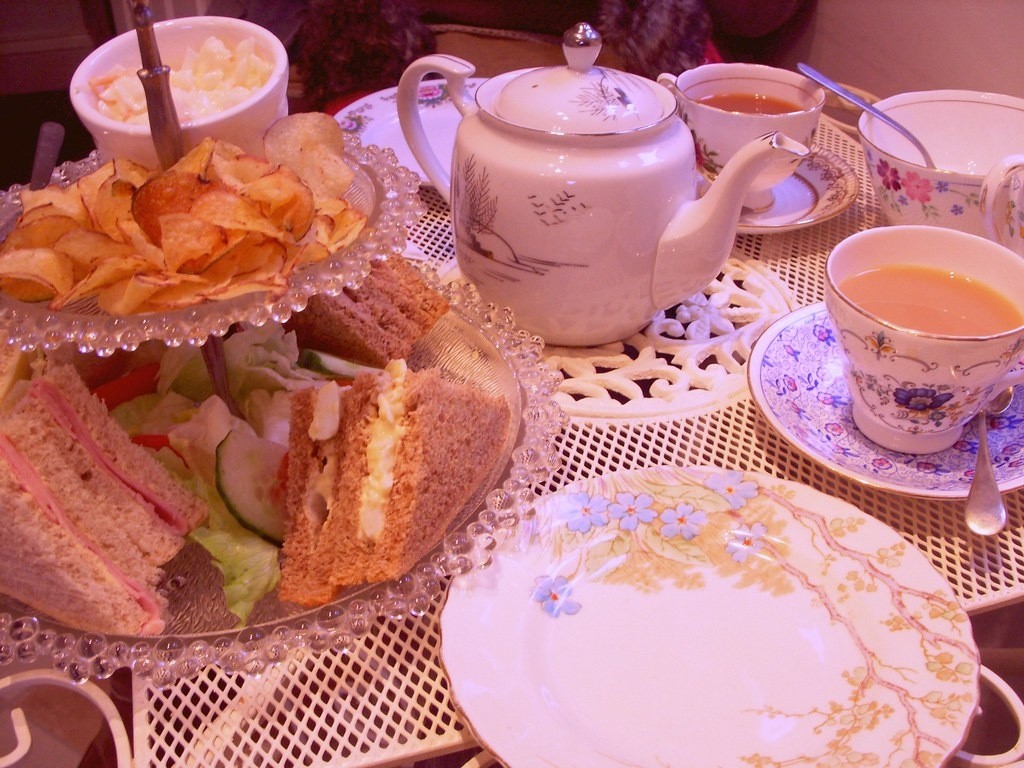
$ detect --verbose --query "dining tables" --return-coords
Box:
[116,111,1021,768]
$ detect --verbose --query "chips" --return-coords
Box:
[0,110,372,318]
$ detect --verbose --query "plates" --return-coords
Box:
[437,462,984,768]
[694,142,860,236]
[0,123,428,357]
[0,267,570,686]
[331,78,489,187]
[747,301,1024,501]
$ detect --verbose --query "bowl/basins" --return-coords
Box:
[856,88,1024,259]
[69,14,289,171]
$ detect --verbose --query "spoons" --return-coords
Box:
[965,385,1013,535]
[795,62,937,168]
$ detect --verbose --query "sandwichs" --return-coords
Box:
[0,251,512,635]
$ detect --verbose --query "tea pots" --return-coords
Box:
[396,23,810,347]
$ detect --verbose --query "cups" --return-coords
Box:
[824,224,1024,455]
[657,62,828,215]
